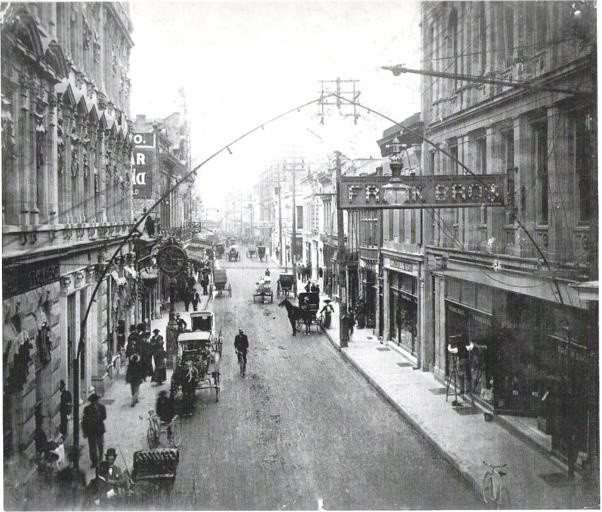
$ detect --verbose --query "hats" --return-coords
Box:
[88,392,101,402]
[104,448,119,457]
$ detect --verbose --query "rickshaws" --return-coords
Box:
[190,311,215,332]
[216,238,268,262]
[254,272,297,303]
[127,448,179,507]
[209,270,232,298]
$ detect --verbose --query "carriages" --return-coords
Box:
[279,293,320,336]
[171,330,223,402]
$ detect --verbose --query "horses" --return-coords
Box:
[174,362,201,416]
[279,298,312,336]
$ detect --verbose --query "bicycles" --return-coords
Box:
[146,408,184,450]
[482,461,512,510]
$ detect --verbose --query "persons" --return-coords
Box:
[175,312,187,330]
[156,390,173,438]
[320,299,335,329]
[104,447,124,492]
[265,267,271,276]
[347,307,354,334]
[58,379,72,440]
[175,263,212,312]
[82,392,106,468]
[296,262,323,294]
[125,325,167,409]
[234,327,249,376]
[80,461,110,505]
